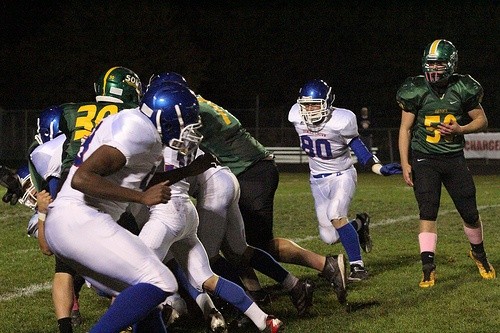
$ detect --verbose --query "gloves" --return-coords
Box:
[379,162,405,177]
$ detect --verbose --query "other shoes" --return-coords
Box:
[209,317,239,333]
[229,291,272,316]
[70,309,83,326]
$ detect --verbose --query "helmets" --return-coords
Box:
[139,81,204,161]
[421,39,459,87]
[35,105,69,144]
[146,72,190,96]
[93,66,144,109]
[296,79,335,128]
[14,166,40,210]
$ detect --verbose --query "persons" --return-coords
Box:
[397,39,496,287]
[287,80,403,280]
[3,67,346,333]
[357,107,373,154]
[46,82,204,333]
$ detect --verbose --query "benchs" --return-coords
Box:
[265,146,378,165]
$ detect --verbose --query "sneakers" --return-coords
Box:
[317,253,347,304]
[356,212,373,253]
[347,264,369,283]
[469,247,496,279]
[418,263,436,288]
[287,276,314,317]
[261,315,282,333]
